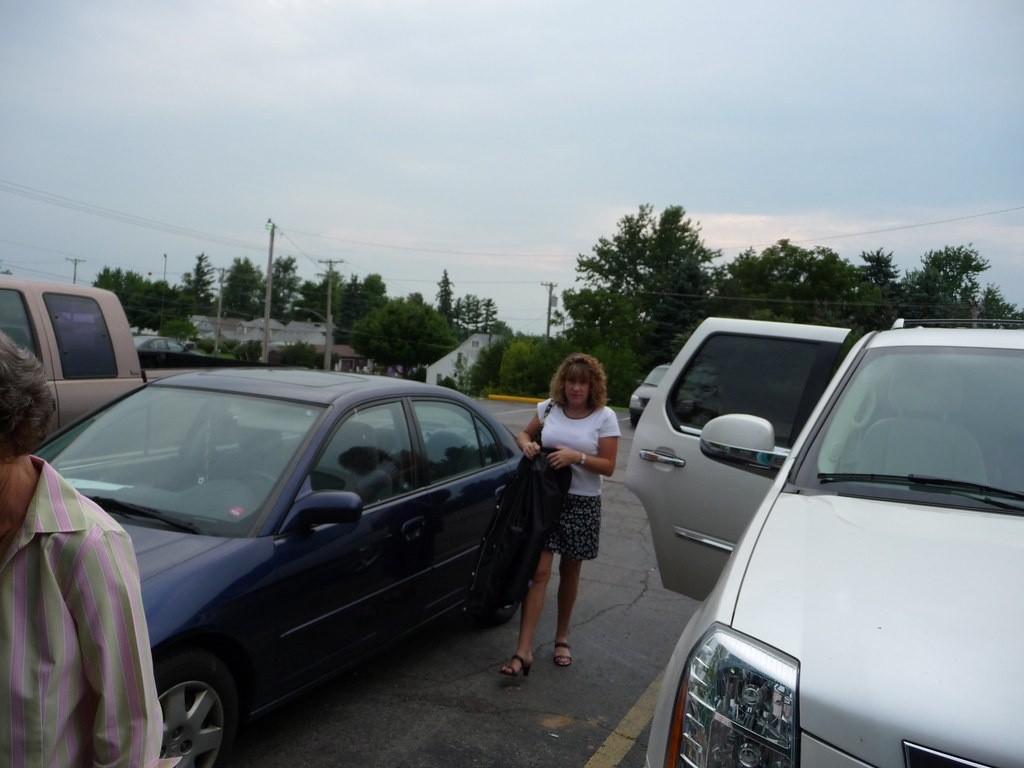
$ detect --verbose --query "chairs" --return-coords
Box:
[319,421,388,506]
[858,368,987,485]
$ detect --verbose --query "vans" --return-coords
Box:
[0,276,147,446]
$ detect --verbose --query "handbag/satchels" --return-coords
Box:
[535,399,555,447]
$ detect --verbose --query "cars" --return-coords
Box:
[32,367,529,768]
[628,363,707,428]
[130,335,197,368]
[623,305,1024,768]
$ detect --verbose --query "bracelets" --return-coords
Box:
[578,454,586,466]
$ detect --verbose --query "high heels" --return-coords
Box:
[500,654,532,676]
[554,643,572,666]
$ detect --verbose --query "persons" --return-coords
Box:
[498,353,622,675]
[0,332,163,768]
